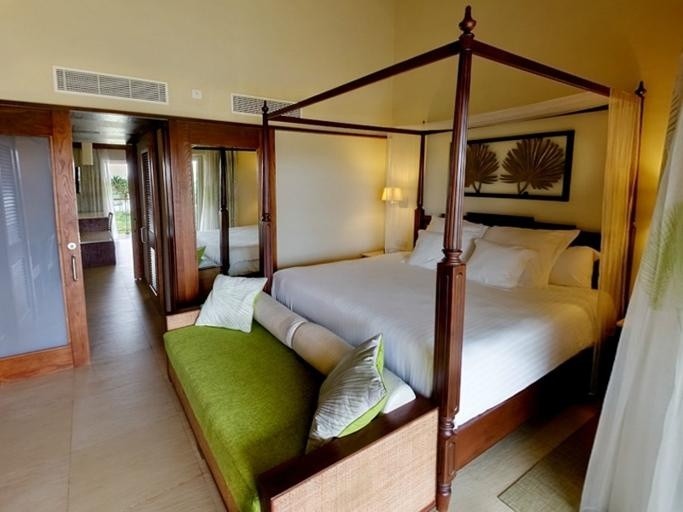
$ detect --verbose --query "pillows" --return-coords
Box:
[400,216,601,292]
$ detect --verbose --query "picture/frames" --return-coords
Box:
[464,129,576,202]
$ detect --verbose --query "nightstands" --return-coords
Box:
[361,248,385,257]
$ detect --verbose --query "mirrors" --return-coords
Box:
[192,145,262,281]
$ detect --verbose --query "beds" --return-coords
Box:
[259,5,647,512]
[196,223,260,277]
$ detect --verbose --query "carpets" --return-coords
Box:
[496,411,600,512]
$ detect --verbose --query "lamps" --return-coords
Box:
[381,187,402,202]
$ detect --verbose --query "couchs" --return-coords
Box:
[162,290,439,512]
